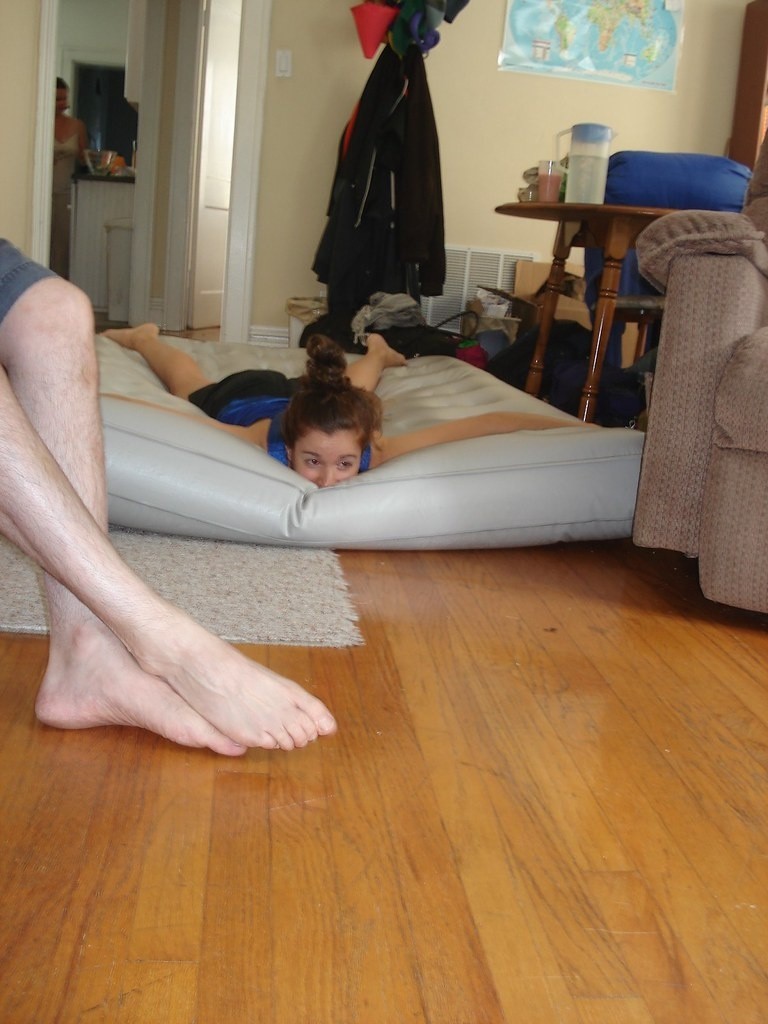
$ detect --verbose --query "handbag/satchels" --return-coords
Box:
[376,311,479,359]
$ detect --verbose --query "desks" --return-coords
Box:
[495,201,679,426]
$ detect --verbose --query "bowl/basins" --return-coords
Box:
[84,149,117,177]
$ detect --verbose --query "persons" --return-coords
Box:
[0,238,338,756]
[101,323,594,486]
[52,77,96,276]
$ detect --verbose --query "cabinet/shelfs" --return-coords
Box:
[67,175,136,313]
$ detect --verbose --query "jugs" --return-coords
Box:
[555,123,620,205]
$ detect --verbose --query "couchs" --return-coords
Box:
[634,132,768,615]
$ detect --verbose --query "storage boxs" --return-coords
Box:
[476,260,640,368]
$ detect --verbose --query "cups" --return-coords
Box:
[538,160,563,203]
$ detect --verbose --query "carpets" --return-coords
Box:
[0,520,367,648]
